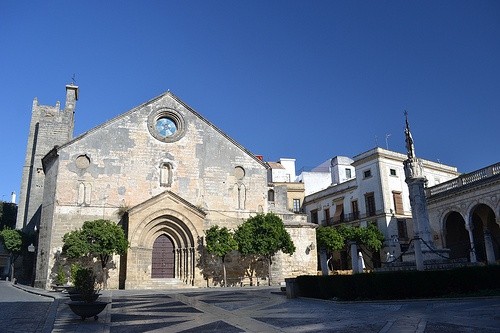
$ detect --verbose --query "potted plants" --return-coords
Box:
[65,290,102,301]
[66,270,96,293]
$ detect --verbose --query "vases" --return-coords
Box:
[65,301,110,321]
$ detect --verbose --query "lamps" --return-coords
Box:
[310,243,315,250]
